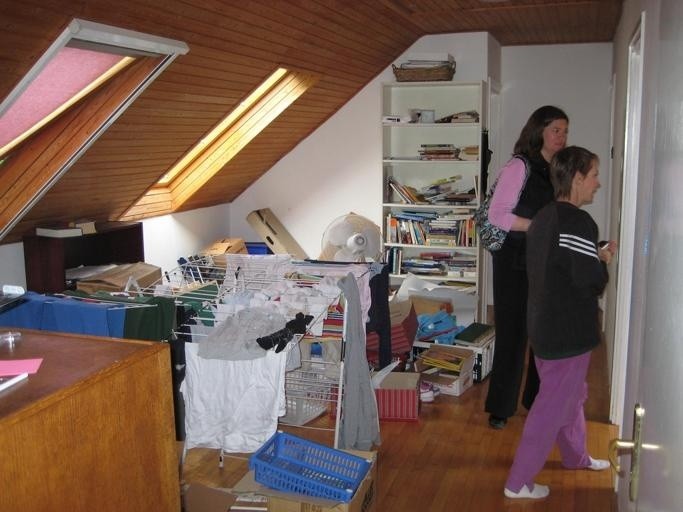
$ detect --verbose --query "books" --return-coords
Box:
[418,323,494,379]
[384,209,477,247]
[383,247,476,278]
[450,111,476,123]
[389,173,478,205]
[418,143,479,160]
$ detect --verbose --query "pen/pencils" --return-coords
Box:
[0,373,29,390]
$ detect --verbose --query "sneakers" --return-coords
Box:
[419,379,436,403]
[488,413,507,429]
[586,457,611,471]
[504,483,550,499]
[432,387,441,397]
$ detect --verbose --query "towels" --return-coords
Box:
[1,290,176,340]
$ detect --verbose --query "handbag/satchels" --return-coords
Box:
[473,154,531,252]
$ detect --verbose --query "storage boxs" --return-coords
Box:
[329,372,421,421]
[415,345,474,397]
[199,239,249,269]
[76,262,162,296]
[228,449,378,511]
[366,300,419,362]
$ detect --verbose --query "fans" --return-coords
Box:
[321,214,382,263]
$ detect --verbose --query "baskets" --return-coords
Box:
[391,61,456,82]
[248,431,373,503]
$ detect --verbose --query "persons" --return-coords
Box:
[503,145,610,500]
[485,105,569,429]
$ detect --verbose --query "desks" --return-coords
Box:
[0,326,182,512]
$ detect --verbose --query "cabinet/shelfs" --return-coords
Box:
[23,220,145,291]
[380,80,486,325]
[123,253,372,450]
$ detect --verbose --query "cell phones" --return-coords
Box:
[598,240,610,249]
